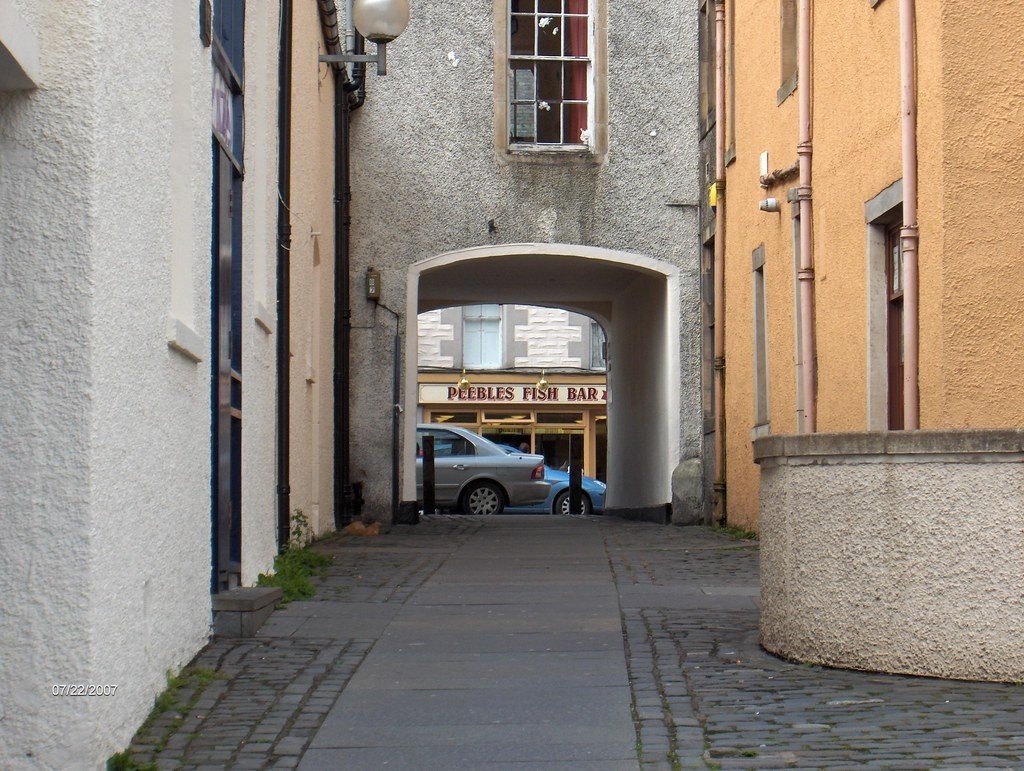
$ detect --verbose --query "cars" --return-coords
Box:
[434,443,606,515]
[416,423,551,516]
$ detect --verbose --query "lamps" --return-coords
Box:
[318,0,411,76]
